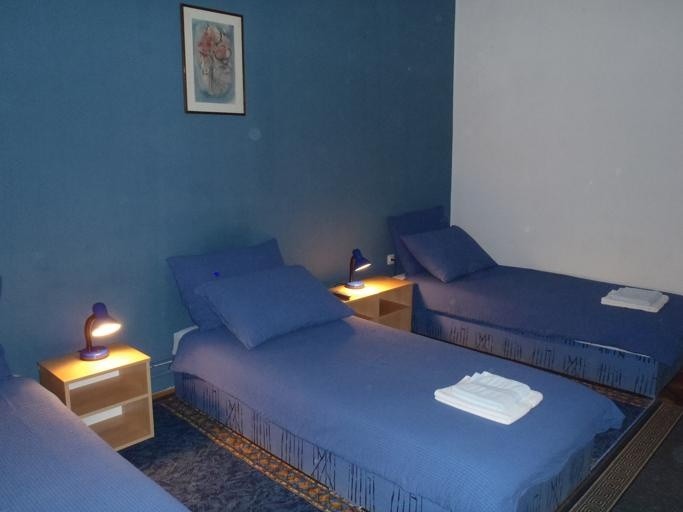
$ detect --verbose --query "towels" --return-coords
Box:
[435,372,543,424]
[601,285,670,313]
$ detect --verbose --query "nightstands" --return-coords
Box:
[330,275,412,333]
[37,343,156,452]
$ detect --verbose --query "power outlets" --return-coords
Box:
[386,254,397,265]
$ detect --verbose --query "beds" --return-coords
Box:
[393,265,682,399]
[173,316,625,512]
[0,377,188,512]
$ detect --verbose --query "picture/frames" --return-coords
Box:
[180,4,247,115]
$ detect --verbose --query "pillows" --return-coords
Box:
[402,227,499,282]
[196,266,355,350]
[388,207,450,273]
[0,347,13,377]
[169,238,286,333]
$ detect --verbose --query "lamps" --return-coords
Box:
[348,249,371,287]
[80,302,122,362]
